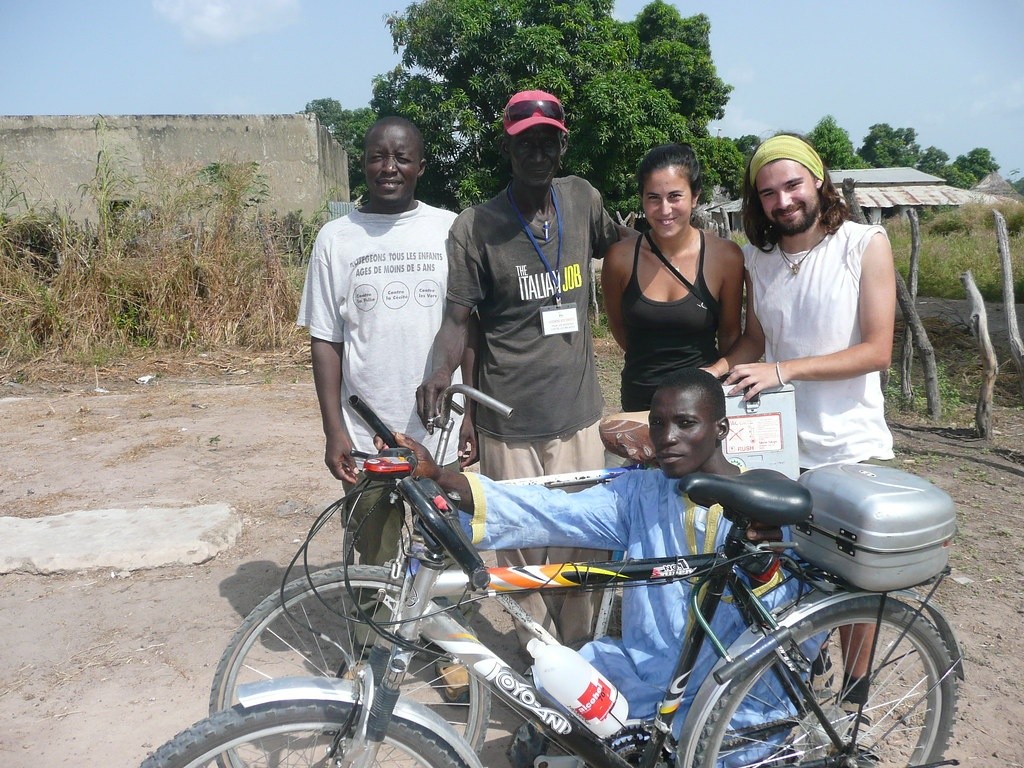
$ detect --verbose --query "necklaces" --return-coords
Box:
[515,187,553,243]
[652,237,692,271]
[778,228,829,274]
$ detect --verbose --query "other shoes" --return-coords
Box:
[435,662,471,705]
[343,656,368,681]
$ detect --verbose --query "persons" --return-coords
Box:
[373,368,828,768]
[298,90,898,764]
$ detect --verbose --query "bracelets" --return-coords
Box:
[776,361,786,387]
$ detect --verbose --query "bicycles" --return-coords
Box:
[135,382,969,768]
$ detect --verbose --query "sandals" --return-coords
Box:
[804,664,835,692]
[840,701,880,764]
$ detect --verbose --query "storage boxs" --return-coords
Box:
[722,382,799,480]
[788,463,957,591]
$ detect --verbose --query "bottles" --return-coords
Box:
[526,638,629,740]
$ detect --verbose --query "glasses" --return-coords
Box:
[504,99,566,122]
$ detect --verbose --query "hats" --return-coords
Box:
[502,90,568,135]
